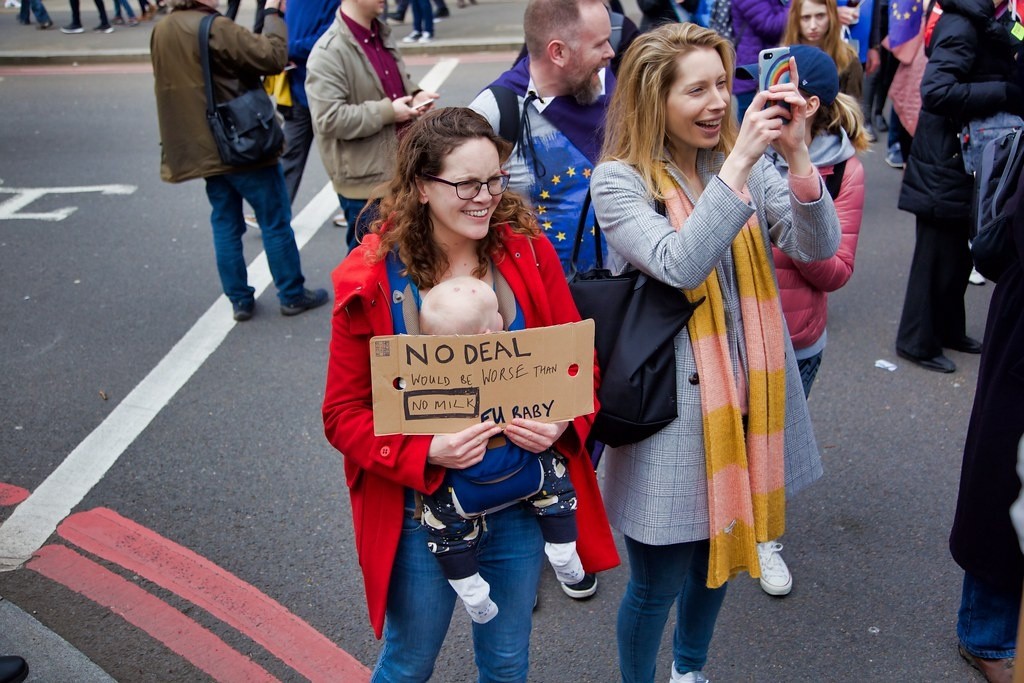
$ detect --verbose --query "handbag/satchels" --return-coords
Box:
[198,12,284,165]
[565,189,706,451]
[956,112,1024,177]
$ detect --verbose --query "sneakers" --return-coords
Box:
[668,659,714,683]
[756,540,793,595]
[333,210,349,228]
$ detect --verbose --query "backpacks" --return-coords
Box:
[967,129,1024,282]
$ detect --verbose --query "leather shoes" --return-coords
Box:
[946,336,983,354]
[918,355,956,373]
[959,640,1014,683]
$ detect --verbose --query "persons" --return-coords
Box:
[151,0,440,321]
[603,0,944,167]
[895,0,1024,374]
[4,0,168,34]
[947,165,1024,683]
[735,45,865,597]
[465,0,619,612]
[385,0,477,44]
[590,23,842,683]
[381,276,585,624]
[323,107,621,683]
[0,656,30,683]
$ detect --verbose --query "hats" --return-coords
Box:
[790,45,839,105]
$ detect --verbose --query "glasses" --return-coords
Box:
[416,168,511,199]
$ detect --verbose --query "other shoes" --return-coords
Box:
[863,118,878,142]
[233,303,254,321]
[885,142,904,167]
[279,287,328,316]
[875,112,888,131]
[244,210,261,228]
[387,2,474,43]
[561,571,598,598]
[969,266,986,286]
[14,0,159,34]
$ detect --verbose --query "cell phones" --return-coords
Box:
[758,46,794,125]
[412,98,435,110]
